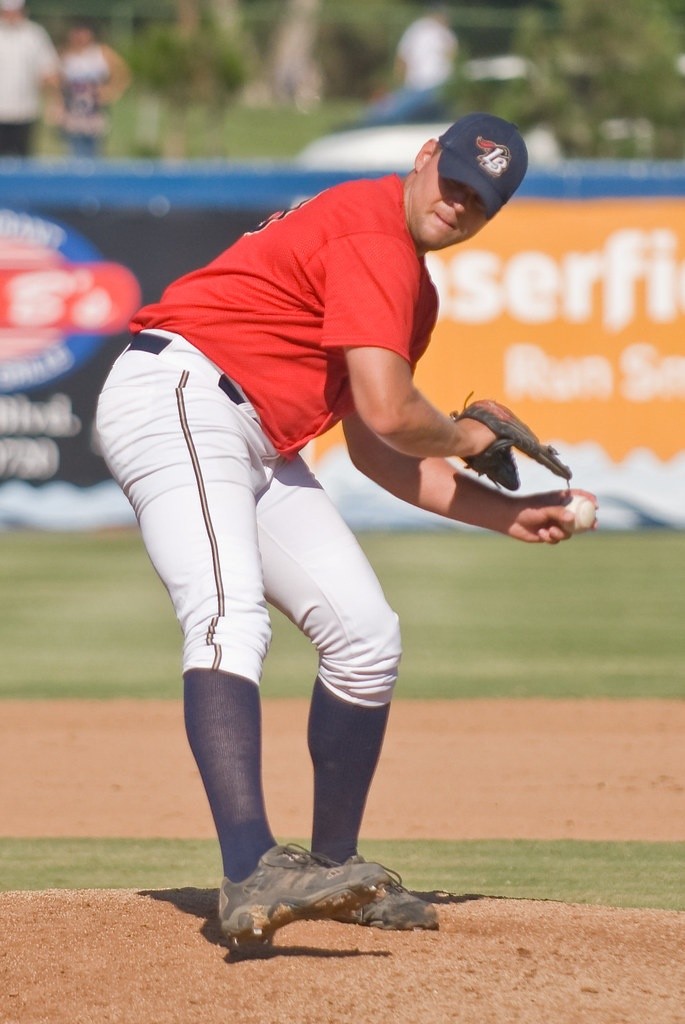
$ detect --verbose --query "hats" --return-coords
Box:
[437,112,529,219]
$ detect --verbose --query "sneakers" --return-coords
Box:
[212,843,395,957]
[331,855,442,931]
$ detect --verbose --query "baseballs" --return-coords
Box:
[558,494,596,534]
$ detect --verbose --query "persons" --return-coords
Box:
[0,0,459,159]
[97,111,598,945]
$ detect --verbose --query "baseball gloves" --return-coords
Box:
[449,391,572,492]
